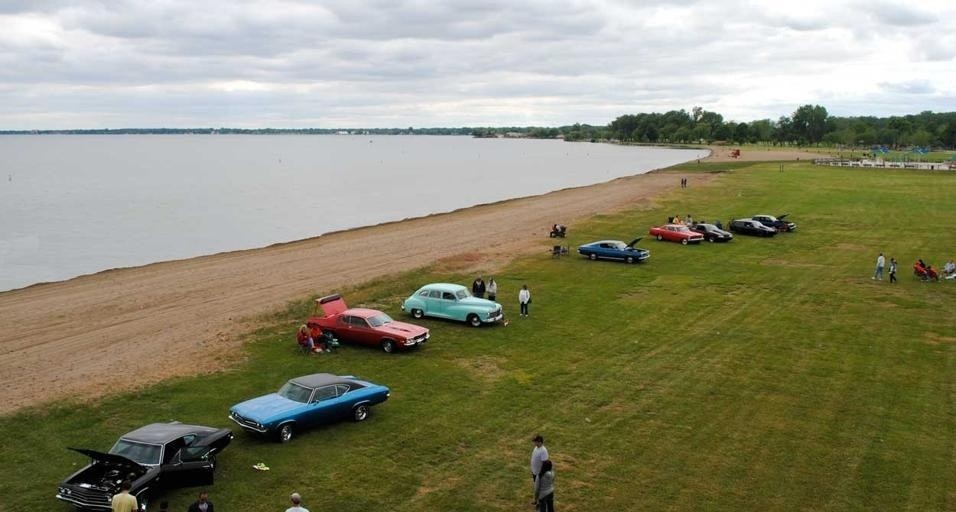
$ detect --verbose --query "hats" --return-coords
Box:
[532,435,545,443]
[290,491,302,504]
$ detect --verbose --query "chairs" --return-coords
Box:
[296,337,311,357]
[552,245,561,258]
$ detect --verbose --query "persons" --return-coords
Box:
[486,278,496,300]
[889,258,896,282]
[188,492,214,512]
[673,214,694,230]
[312,323,331,352]
[531,436,549,504]
[286,493,309,512]
[111,482,138,512]
[296,324,316,354]
[871,254,885,280]
[518,284,530,317]
[472,275,485,298]
[534,460,555,511]
[550,224,566,237]
[681,178,686,188]
[915,259,955,281]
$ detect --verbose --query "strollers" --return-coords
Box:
[911,259,938,283]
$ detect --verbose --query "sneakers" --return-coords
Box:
[311,345,317,351]
[519,313,528,318]
[871,277,897,284]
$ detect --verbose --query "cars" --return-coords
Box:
[225,371,393,447]
[580,234,651,265]
[304,294,429,355]
[400,283,505,330]
[649,214,799,246]
[56,420,234,508]
